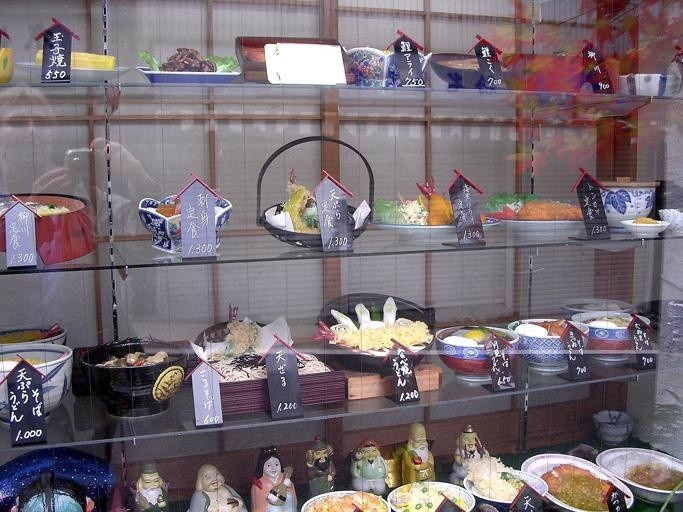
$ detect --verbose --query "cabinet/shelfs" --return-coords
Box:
[2,65,683,472]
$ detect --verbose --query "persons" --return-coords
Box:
[350,441,386,494]
[134,460,169,510]
[2,82,165,339]
[250,447,297,512]
[401,423,436,484]
[449,424,487,486]
[189,463,247,512]
[303,434,338,498]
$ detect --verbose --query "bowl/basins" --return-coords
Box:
[660,206,683,233]
[138,195,234,253]
[621,220,673,237]
[315,290,433,373]
[429,52,511,89]
[340,45,433,88]
[434,294,638,381]
[298,466,554,512]
[0,321,191,427]
[620,73,679,97]
[591,409,635,445]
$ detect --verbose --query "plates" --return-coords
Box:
[18,59,130,87]
[370,216,496,241]
[522,447,682,512]
[491,217,591,238]
[136,63,244,83]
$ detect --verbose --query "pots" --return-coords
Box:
[591,180,658,230]
